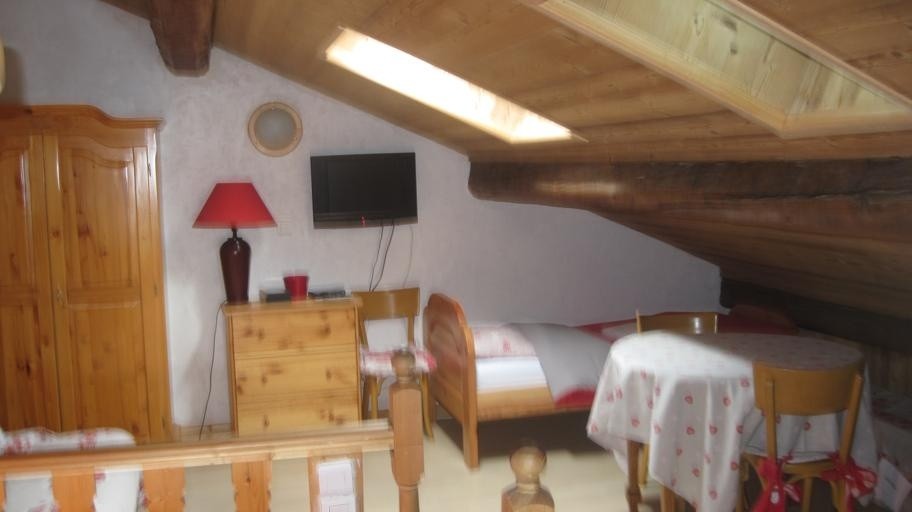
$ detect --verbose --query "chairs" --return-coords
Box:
[736,357,868,512]
[352,288,436,440]
[634,308,720,486]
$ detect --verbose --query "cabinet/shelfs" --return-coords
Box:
[223,298,361,437]
[0,106,171,450]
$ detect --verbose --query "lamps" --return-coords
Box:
[192,181,278,306]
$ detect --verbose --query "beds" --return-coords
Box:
[421,292,799,466]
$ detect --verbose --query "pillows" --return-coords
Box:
[469,323,537,360]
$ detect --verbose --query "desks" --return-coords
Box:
[606,328,866,510]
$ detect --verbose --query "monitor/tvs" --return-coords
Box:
[311,152,418,230]
